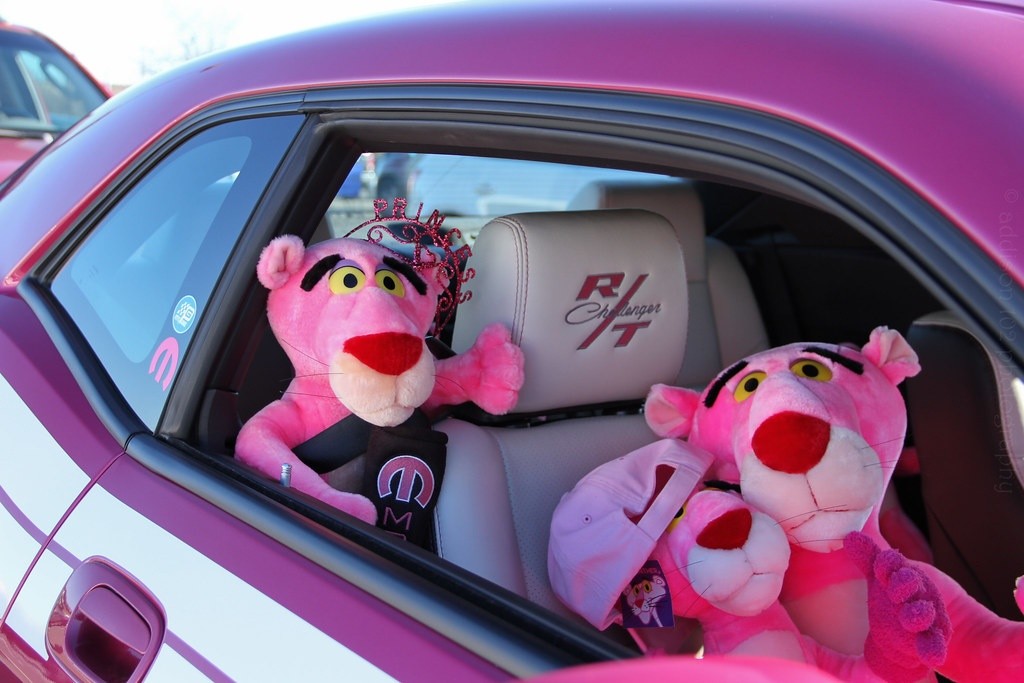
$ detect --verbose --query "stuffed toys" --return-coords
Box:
[236,234,525,526]
[644,325,1024,683]
[644,466,951,682]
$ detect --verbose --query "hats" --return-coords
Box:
[549,439,714,630]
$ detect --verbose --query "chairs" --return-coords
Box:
[572,180,769,392]
[435,209,691,628]
[903,311,1024,622]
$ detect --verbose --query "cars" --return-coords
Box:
[0,1,1024,683]
[0,20,114,184]
